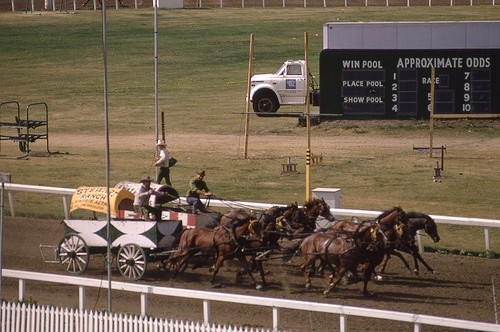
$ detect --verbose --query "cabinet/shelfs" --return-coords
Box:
[0,100,59,159]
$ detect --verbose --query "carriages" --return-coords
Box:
[39,162,441,298]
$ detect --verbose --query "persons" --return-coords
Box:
[133,175,169,221]
[154,139,173,187]
[186,171,217,216]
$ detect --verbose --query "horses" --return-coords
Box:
[155,197,440,297]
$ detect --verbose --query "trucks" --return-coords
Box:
[250,22,500,121]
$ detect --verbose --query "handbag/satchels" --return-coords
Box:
[169,157,177,168]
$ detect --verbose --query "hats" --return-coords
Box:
[139,176,153,183]
[195,170,206,176]
[156,139,167,146]
